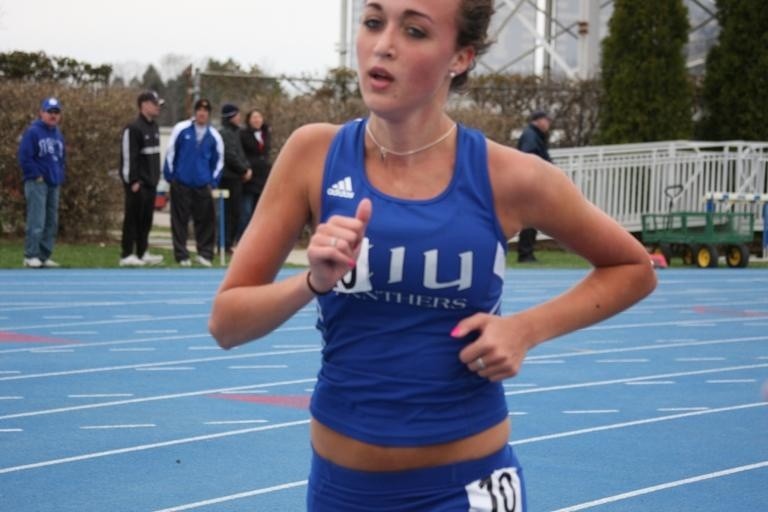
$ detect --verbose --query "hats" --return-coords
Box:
[527,111,545,120]
[223,105,239,118]
[195,98,211,112]
[137,92,164,106]
[43,97,61,111]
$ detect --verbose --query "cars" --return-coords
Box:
[153,123,180,213]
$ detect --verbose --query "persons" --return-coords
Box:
[18,98,66,267]
[117,91,272,266]
[208,0,658,511]
[517,112,555,261]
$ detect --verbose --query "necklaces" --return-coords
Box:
[365,123,457,161]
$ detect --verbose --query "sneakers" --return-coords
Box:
[120,252,164,266]
[24,257,58,267]
[181,258,192,266]
[195,255,211,266]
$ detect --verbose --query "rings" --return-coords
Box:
[332,236,336,247]
[477,357,485,370]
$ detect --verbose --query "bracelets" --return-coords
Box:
[306,271,333,296]
